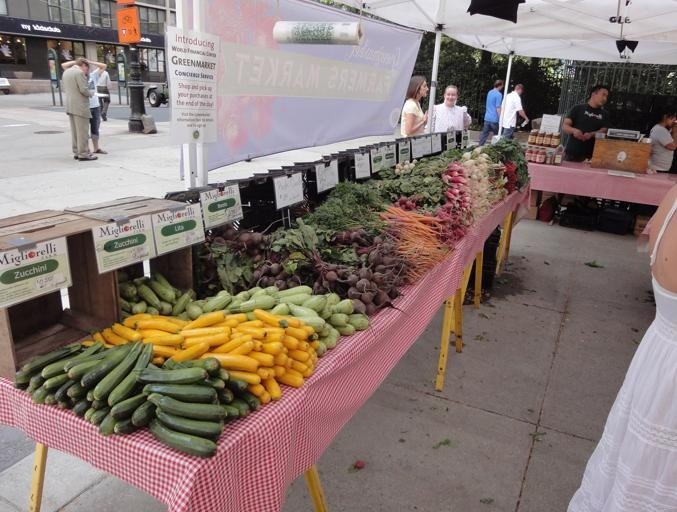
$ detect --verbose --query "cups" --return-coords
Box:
[643,138,651,144]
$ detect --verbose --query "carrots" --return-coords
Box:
[81,308,320,404]
[376,206,450,281]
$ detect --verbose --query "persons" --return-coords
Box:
[432,84,469,133]
[569,182,677,512]
[98,64,111,121]
[561,85,610,161]
[478,79,501,146]
[60,58,108,155]
[400,76,426,136]
[60,58,98,160]
[646,111,675,173]
[501,85,528,144]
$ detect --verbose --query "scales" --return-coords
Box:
[607,128,641,140]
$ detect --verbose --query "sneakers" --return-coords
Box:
[101,113,108,121]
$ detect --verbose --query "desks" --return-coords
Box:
[517,156,677,208]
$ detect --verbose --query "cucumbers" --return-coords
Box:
[15,338,259,458]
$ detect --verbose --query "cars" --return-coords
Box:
[146,79,169,109]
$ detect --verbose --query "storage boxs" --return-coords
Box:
[521,184,654,238]
[589,132,655,175]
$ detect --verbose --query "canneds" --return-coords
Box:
[525,129,563,166]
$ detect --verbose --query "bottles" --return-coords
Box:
[520,119,528,127]
[546,144,563,166]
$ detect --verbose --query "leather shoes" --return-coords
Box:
[74,154,98,160]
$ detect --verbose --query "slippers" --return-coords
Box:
[93,148,109,154]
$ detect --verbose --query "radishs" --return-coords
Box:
[461,145,493,220]
[394,157,417,176]
